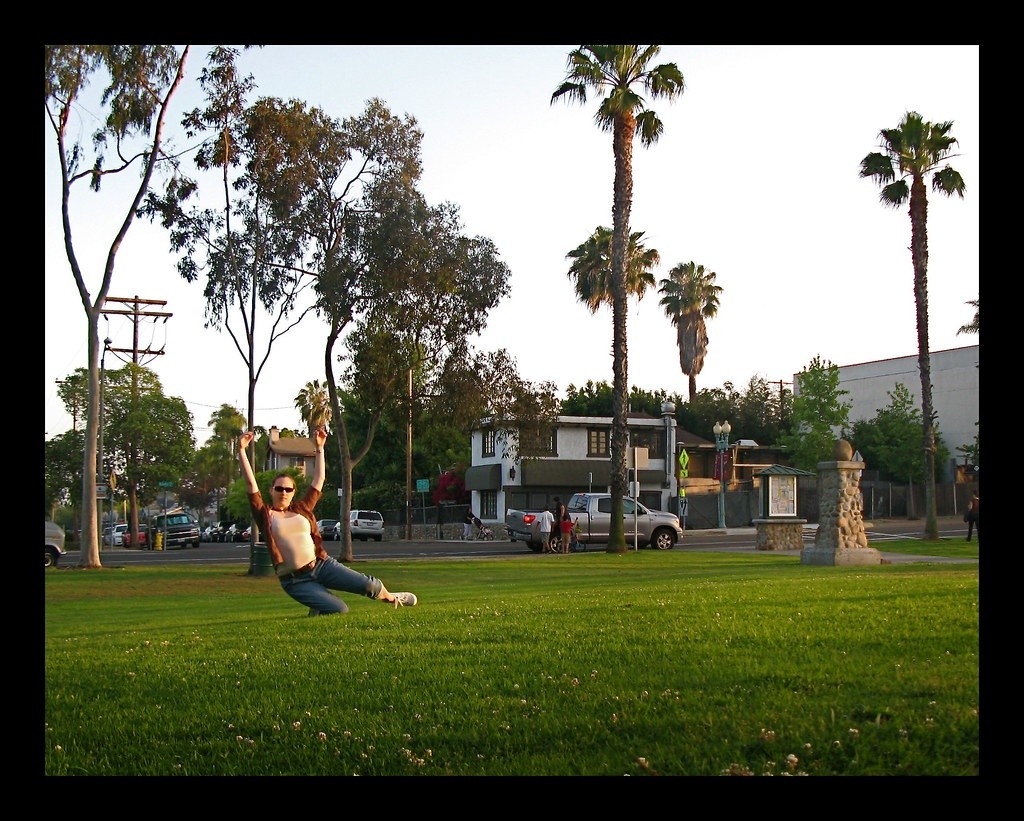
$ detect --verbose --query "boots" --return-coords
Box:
[375,579,417,609]
[308,608,320,616]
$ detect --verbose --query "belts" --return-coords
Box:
[283,560,316,579]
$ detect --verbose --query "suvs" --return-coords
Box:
[145,511,201,550]
[333,510,385,541]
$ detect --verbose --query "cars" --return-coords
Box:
[45,521,69,567]
[102,520,262,548]
[317,519,339,540]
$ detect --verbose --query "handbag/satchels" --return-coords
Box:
[963,510,971,522]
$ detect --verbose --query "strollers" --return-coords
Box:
[470,516,497,541]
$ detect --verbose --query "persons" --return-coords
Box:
[237,426,419,615]
[967,490,979,542]
[461,508,475,541]
[534,496,579,554]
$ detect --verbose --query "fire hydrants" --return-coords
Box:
[153,529,164,551]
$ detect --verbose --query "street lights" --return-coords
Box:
[98,337,113,553]
[713,420,732,528]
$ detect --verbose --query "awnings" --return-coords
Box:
[465,464,502,490]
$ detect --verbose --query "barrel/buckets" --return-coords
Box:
[252,542,276,577]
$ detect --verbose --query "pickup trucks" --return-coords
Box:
[502,491,684,554]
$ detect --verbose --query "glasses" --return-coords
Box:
[273,486,294,492]
